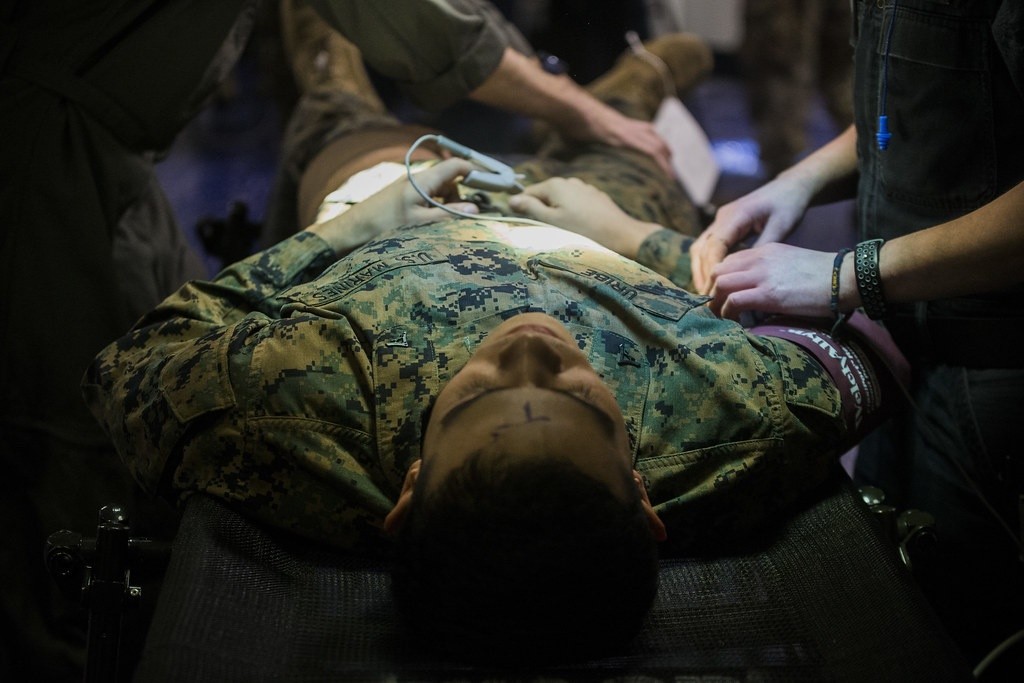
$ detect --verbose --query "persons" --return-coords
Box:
[0,0,671,682]
[738,0,859,175]
[87,2,918,682]
[686,0,1024,683]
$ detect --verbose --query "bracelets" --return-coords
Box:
[854,236,890,321]
[832,246,852,317]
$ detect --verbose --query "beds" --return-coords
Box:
[48,153,981,683]
